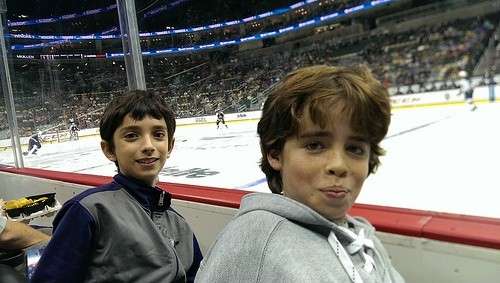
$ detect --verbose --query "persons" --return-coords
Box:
[23,132,45,156]
[69,123,80,140]
[196,0,500,117]
[0,34,195,141]
[30,90,203,283]
[457,70,478,111]
[216,108,227,129]
[194,65,404,283]
